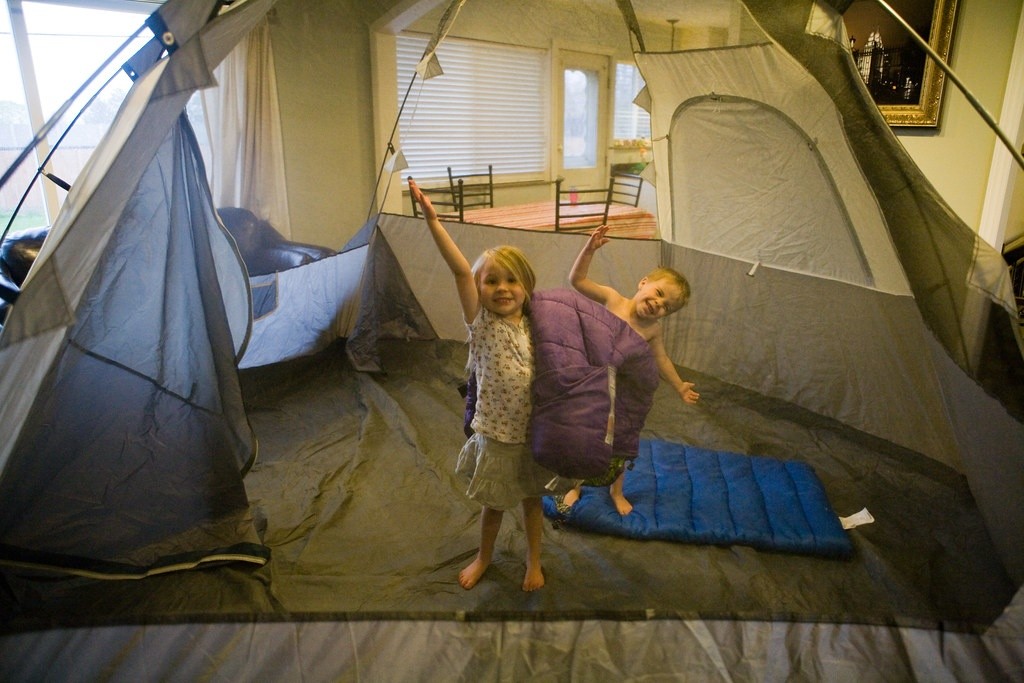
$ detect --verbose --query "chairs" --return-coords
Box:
[408,176,464,222]
[554,178,615,233]
[606,166,643,208]
[448,165,493,212]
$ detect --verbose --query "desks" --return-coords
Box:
[417,199,656,239]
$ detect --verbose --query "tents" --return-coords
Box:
[0,1,1024,683]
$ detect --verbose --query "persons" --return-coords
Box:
[406,181,584,591]
[558,224,701,515]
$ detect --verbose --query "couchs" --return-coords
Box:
[0,224,51,335]
[215,206,337,283]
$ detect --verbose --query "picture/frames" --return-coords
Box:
[837,0,961,128]
